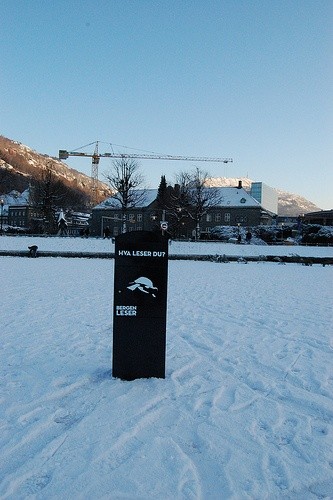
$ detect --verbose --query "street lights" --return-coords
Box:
[0,199,4,232]
[237,223,241,241]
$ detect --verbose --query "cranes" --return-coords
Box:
[59,141,233,215]
